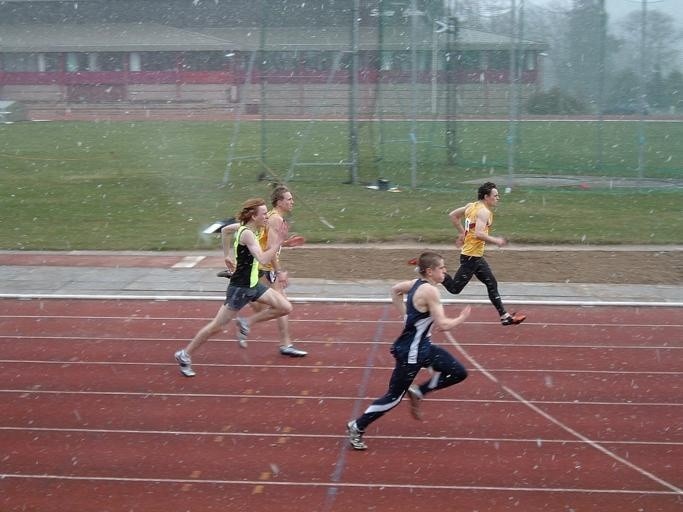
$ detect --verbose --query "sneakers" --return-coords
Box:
[235,318,250,349]
[174,349,197,377]
[346,419,369,451]
[501,313,526,326]
[407,384,426,422]
[280,344,308,358]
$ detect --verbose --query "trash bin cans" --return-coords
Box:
[230,85,239,103]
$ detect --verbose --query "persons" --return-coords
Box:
[347,253,473,451]
[216,185,308,356]
[174,198,292,375]
[409,183,526,325]
